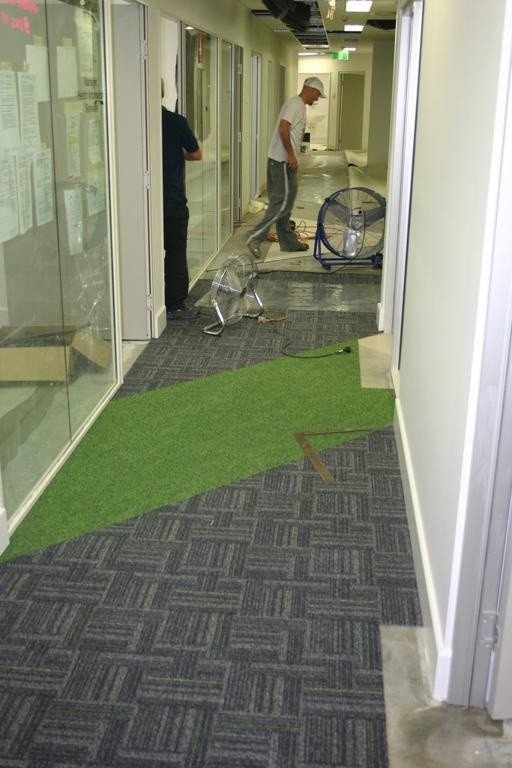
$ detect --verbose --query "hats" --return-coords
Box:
[304,78,327,98]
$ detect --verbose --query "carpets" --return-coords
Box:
[0,332,397,563]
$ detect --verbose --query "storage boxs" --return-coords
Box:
[0,323,112,383]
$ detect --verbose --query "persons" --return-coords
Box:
[243,74,329,260]
[159,77,203,321]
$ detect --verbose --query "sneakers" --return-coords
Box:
[167,304,202,319]
[280,242,309,252]
[241,232,261,259]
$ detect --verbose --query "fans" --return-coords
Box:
[202,252,265,336]
[313,186,387,272]
